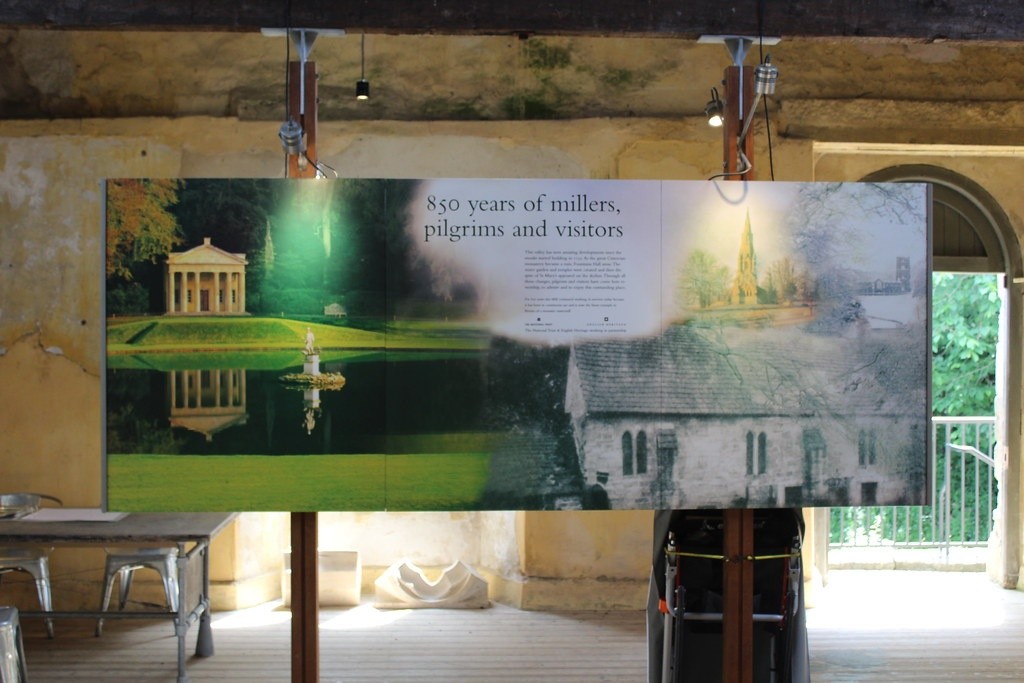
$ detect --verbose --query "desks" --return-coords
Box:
[0,507,237,683]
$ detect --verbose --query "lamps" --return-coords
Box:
[280,115,307,172]
[354,34,370,101]
[737,54,776,149]
[706,88,723,127]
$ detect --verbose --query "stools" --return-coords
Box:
[0,604,28,683]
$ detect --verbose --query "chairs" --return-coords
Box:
[0,492,63,637]
[94,541,179,637]
